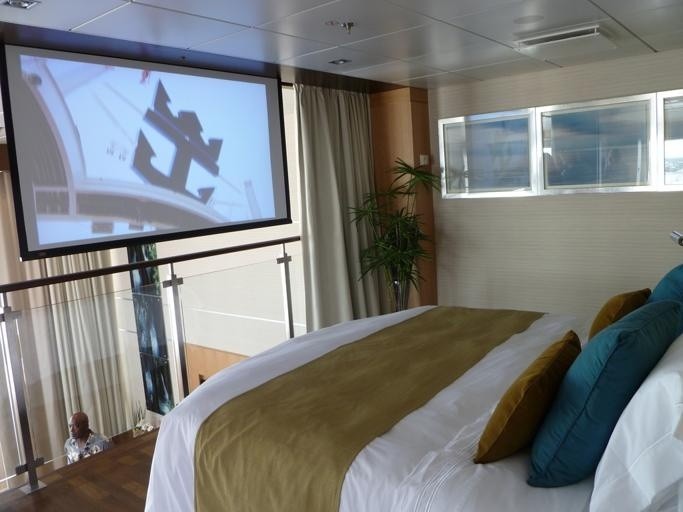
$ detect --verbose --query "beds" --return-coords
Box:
[144,304,683,512]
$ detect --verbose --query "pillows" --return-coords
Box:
[525,303,682,489]
[588,287,652,341]
[587,336,682,510]
[473,328,583,467]
[648,261,682,315]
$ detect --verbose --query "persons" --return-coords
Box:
[62,411,113,466]
